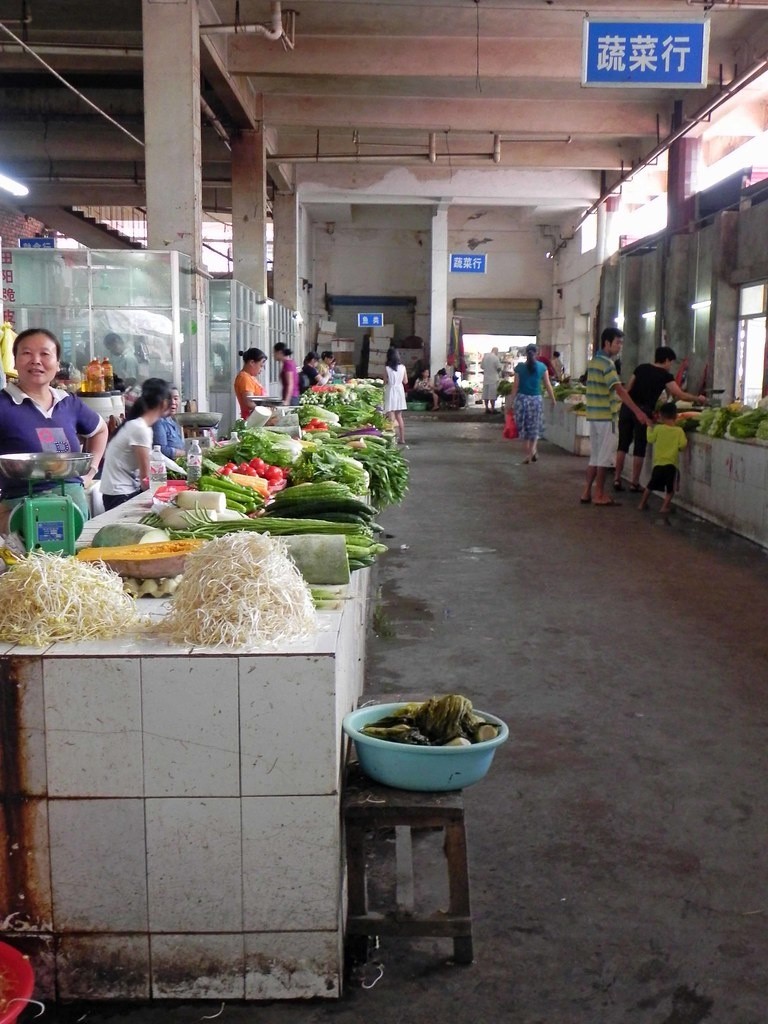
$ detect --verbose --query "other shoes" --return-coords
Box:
[523,459,529,463]
[532,454,537,461]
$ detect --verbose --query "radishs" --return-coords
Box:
[158,490,250,527]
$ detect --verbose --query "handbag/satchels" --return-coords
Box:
[503,410,518,438]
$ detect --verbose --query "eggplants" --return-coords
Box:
[339,428,384,437]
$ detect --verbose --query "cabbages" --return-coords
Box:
[296,405,341,426]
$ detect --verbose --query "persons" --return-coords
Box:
[0,327,108,537]
[480,347,501,414]
[102,332,136,386]
[99,378,172,512]
[299,351,337,395]
[233,347,271,420]
[430,368,456,411]
[578,327,652,506]
[412,369,434,402]
[636,403,688,513]
[270,341,299,406]
[502,344,556,464]
[612,347,710,491]
[381,348,408,444]
[550,351,566,381]
[148,385,190,458]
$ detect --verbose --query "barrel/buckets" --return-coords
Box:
[109,390,125,422]
[76,392,114,427]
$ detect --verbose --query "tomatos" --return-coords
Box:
[302,417,328,431]
[218,456,291,487]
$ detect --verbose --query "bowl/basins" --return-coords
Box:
[344,702,508,791]
[406,402,428,410]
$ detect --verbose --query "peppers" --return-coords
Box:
[187,468,266,514]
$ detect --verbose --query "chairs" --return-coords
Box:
[443,393,460,411]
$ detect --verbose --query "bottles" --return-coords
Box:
[81,357,113,392]
[150,445,168,506]
[230,432,240,445]
[187,440,202,486]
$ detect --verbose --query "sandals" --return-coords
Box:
[614,479,625,491]
[630,484,646,492]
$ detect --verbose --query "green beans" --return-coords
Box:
[165,499,376,541]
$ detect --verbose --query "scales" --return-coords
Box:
[247,394,283,407]
[331,373,346,384]
[0,450,94,559]
[693,388,726,408]
[173,412,224,453]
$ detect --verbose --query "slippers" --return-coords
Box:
[581,495,591,502]
[595,496,623,506]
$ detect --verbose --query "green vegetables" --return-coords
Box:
[330,388,385,433]
[359,694,504,748]
[204,418,293,466]
[167,456,221,480]
[676,402,768,440]
[285,436,413,513]
[497,379,586,411]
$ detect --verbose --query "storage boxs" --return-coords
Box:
[368,323,424,379]
[315,320,356,377]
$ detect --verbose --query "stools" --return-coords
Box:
[342,690,473,979]
